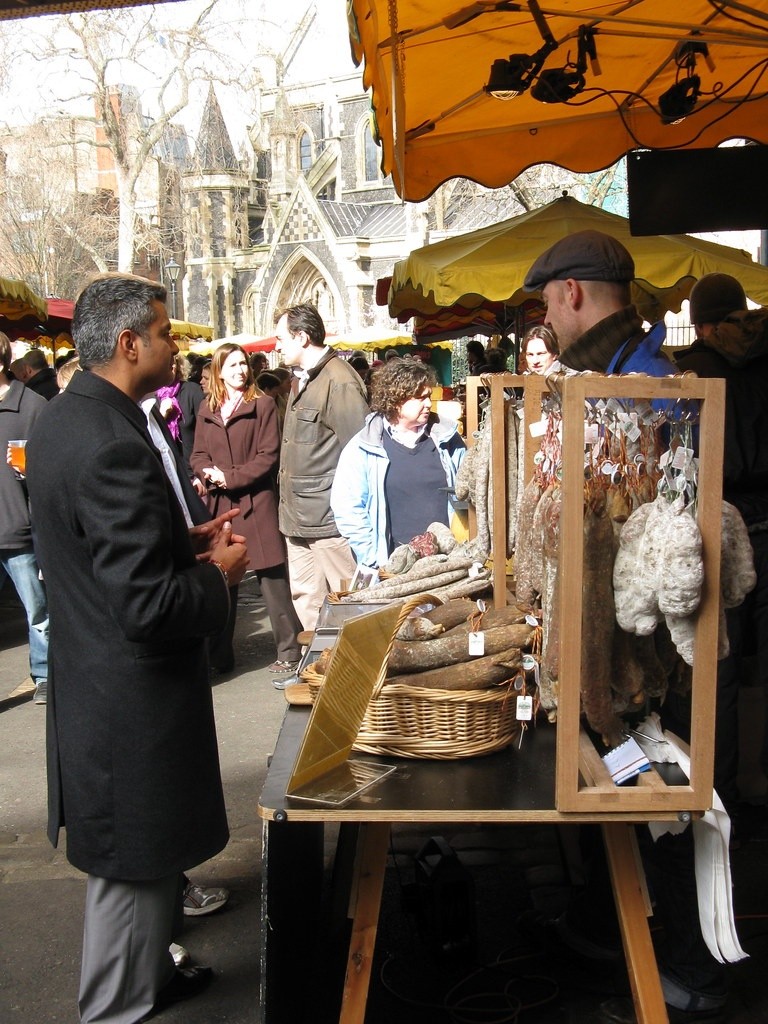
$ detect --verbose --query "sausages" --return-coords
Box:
[318,404,676,739]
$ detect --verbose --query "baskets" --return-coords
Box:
[299,595,524,759]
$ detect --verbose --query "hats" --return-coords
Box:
[689,272,747,324]
[522,229,635,293]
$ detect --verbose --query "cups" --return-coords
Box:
[7,440,27,474]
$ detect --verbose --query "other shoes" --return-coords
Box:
[272,673,299,689]
[157,965,212,1002]
[209,667,221,678]
[268,658,299,674]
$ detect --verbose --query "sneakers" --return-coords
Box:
[33,683,47,704]
[180,884,229,915]
[167,943,190,966]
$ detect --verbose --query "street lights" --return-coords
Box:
[164,254,182,320]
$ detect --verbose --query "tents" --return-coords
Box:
[346,0,768,207]
[325,327,454,387]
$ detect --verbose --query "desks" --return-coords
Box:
[257,598,702,1024]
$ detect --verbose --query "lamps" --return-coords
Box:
[486,54,543,101]
[658,75,700,125]
[531,67,584,103]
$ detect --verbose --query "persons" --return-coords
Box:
[25,273,247,1024]
[0,304,556,703]
[526,228,732,1024]
[672,274,768,970]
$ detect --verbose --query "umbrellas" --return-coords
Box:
[39,291,75,321]
[191,332,281,353]
[168,316,215,338]
[0,274,46,322]
[377,189,768,397]
